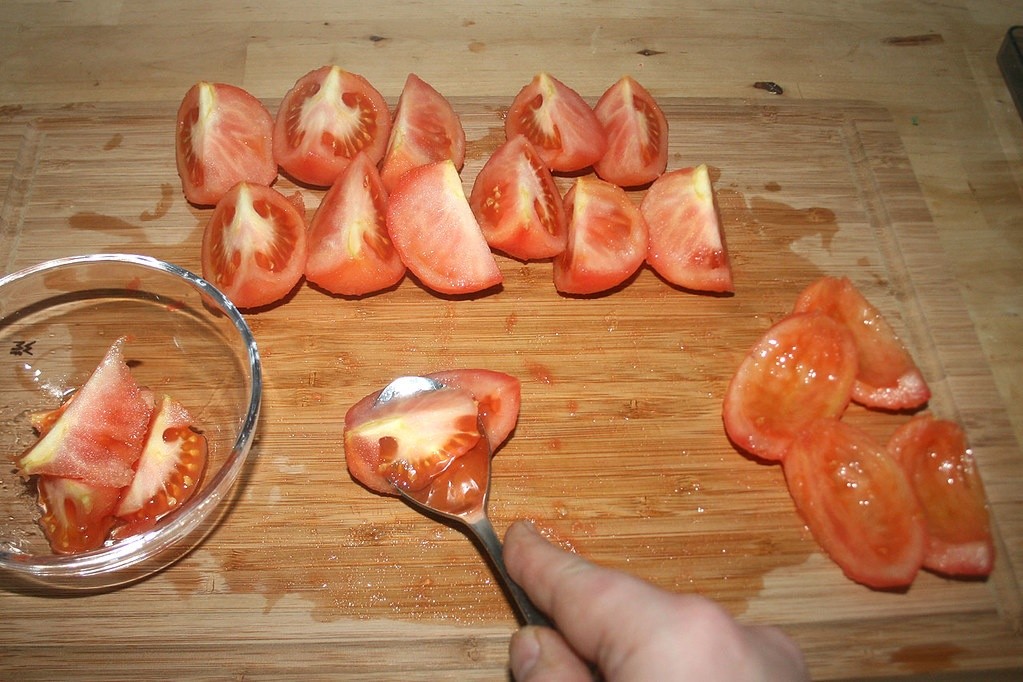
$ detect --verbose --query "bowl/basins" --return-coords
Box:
[1,249,261,587]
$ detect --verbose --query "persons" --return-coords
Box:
[504,514,806,681]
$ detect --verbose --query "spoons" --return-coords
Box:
[370,376,565,640]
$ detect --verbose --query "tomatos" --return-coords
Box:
[14,63,998,589]
